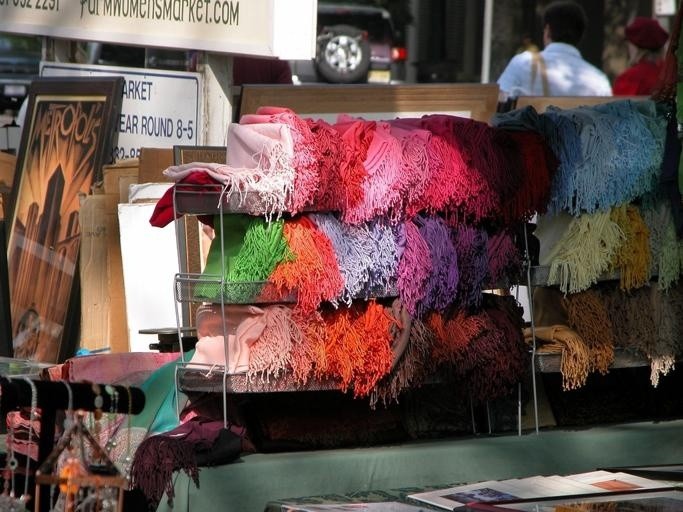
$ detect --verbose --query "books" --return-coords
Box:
[403,466,678,507]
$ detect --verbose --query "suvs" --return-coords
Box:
[291,4,407,85]
[0,32,42,114]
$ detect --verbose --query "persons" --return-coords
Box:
[190,49,296,85]
[493,3,611,101]
[612,18,678,97]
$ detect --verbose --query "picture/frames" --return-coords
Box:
[4,76,125,364]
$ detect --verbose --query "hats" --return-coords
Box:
[624,17,669,50]
